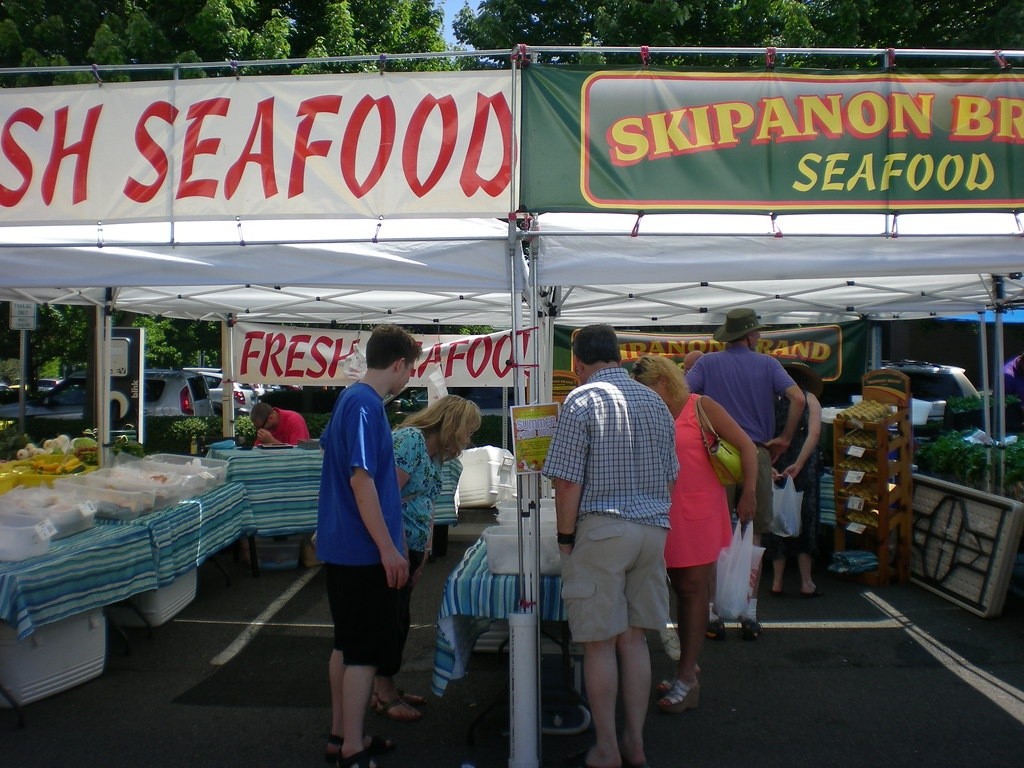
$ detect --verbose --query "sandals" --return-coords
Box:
[325,733,394,763]
[335,746,384,768]
[372,694,424,722]
[371,687,428,708]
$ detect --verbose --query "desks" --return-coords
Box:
[431,536,592,746]
[205,448,463,578]
[0,481,257,731]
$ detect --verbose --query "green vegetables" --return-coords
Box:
[71,436,144,465]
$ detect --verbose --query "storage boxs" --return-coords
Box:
[254,537,302,571]
[0,454,230,563]
[0,606,105,708]
[912,399,932,425]
[457,444,516,509]
[107,565,197,630]
[481,497,563,576]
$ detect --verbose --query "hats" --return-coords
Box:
[713,308,772,342]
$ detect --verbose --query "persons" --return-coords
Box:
[316,324,422,768]
[684,309,805,640]
[543,323,681,768]
[1004,353,1024,432]
[766,362,824,595]
[634,356,758,713]
[372,394,481,722]
[251,402,310,445]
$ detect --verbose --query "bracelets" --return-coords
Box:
[557,529,576,545]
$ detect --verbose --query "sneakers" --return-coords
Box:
[704,619,726,641]
[741,619,763,640]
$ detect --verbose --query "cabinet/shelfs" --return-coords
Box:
[833,369,913,586]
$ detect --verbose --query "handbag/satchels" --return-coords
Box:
[711,516,769,626]
[694,395,758,484]
[769,474,805,538]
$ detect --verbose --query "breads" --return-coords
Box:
[836,398,892,527]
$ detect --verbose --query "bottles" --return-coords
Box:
[190,436,198,455]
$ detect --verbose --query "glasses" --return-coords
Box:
[253,413,271,429]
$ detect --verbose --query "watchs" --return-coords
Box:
[427,547,432,555]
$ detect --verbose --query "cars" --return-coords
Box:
[0,365,303,417]
[394,387,513,417]
[843,359,981,424]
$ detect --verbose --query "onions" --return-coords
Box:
[17,434,75,459]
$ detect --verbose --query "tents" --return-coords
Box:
[1,238,1024,494]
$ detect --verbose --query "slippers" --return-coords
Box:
[771,585,787,597]
[561,746,648,768]
[799,583,822,597]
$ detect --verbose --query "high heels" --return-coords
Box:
[655,679,700,712]
[656,669,700,694]
